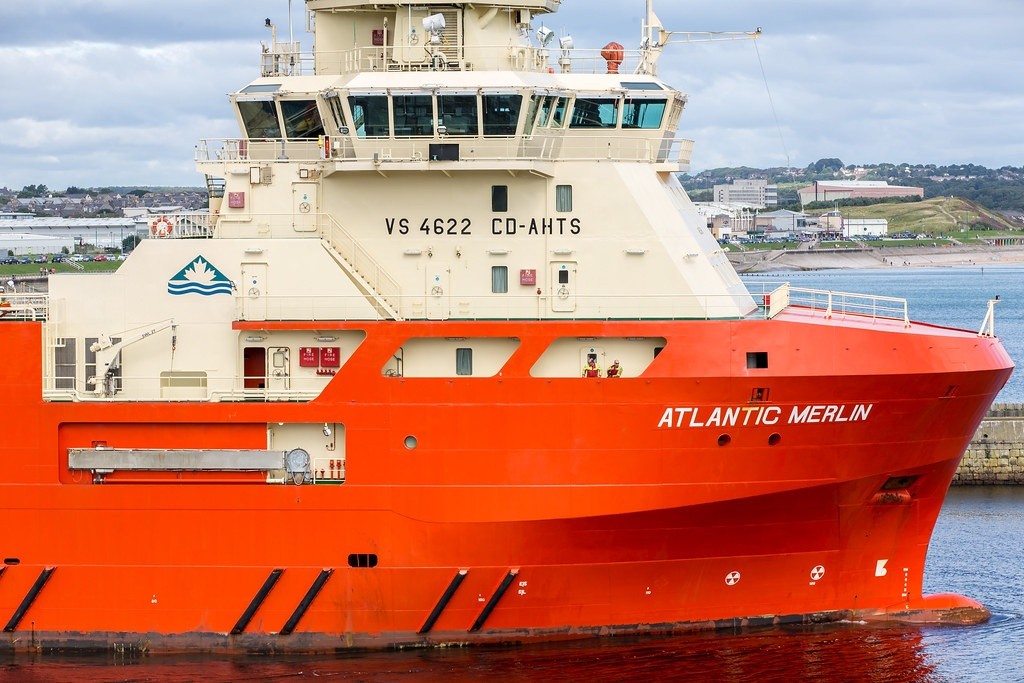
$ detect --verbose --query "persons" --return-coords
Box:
[581,358,603,378]
[0,297,12,313]
[606,360,623,378]
[39,266,55,276]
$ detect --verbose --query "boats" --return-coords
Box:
[0,0,1014,650]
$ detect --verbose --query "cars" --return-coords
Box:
[52,253,67,262]
[0,256,20,264]
[69,253,83,262]
[34,256,48,263]
[854,235,882,240]
[718,236,836,245]
[82,254,116,262]
[18,256,32,264]
[118,254,129,260]
[891,231,917,239]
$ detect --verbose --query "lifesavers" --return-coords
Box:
[153,218,172,237]
